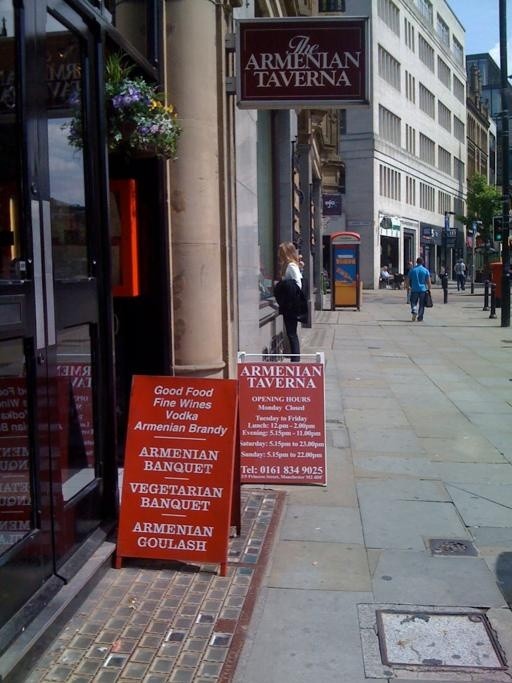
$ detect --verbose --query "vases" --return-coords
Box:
[123,149,157,177]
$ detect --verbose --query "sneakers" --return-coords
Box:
[461,287,465,290]
[418,314,423,321]
[457,288,460,291]
[411,312,417,322]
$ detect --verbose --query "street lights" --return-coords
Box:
[443,210,455,303]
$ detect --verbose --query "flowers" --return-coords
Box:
[61,75,84,153]
[106,58,178,154]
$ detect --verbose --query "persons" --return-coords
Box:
[382,257,483,321]
[273,241,305,362]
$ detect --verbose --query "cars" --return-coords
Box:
[0,254,97,289]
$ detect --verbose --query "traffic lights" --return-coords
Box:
[493,217,501,241]
[477,224,483,233]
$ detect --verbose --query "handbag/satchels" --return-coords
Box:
[425,292,433,307]
